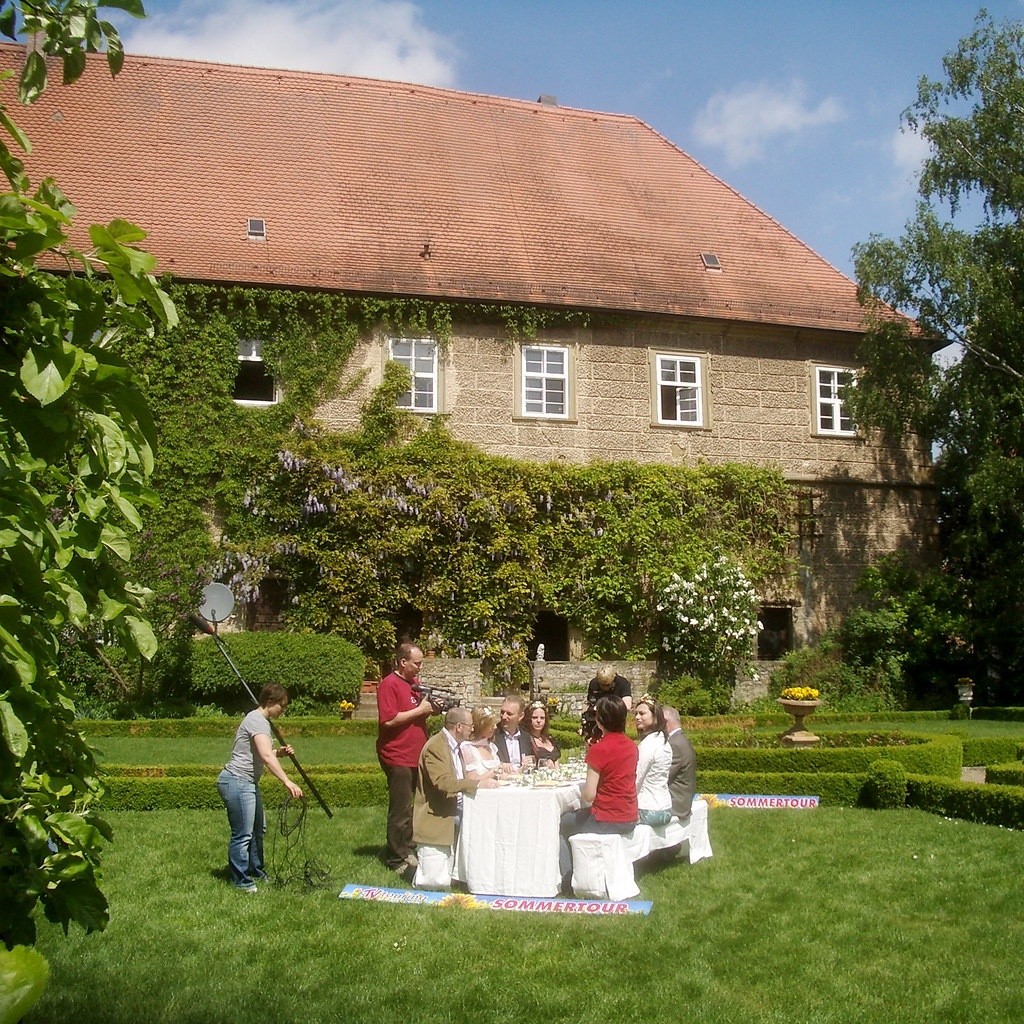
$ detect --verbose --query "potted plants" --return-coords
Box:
[954,677,975,701]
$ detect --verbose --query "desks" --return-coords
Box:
[451,762,595,897]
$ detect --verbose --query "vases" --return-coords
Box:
[342,711,352,719]
[546,704,557,715]
[777,698,819,737]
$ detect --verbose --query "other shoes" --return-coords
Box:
[406,853,418,867]
[394,863,409,874]
[557,889,575,899]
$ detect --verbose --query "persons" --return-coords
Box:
[216,683,303,892]
[373,643,444,874]
[412,705,500,890]
[458,693,559,775]
[636,701,696,862]
[586,665,632,710]
[558,696,640,898]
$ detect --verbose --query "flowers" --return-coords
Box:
[780,686,820,701]
[339,700,355,710]
[547,697,559,704]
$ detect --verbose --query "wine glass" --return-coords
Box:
[492,751,586,787]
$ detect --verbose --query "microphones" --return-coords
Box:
[431,687,456,695]
[188,613,229,648]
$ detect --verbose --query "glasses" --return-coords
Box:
[279,703,285,711]
[463,723,473,731]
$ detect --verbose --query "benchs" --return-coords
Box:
[568,800,714,903]
[416,837,458,892]
